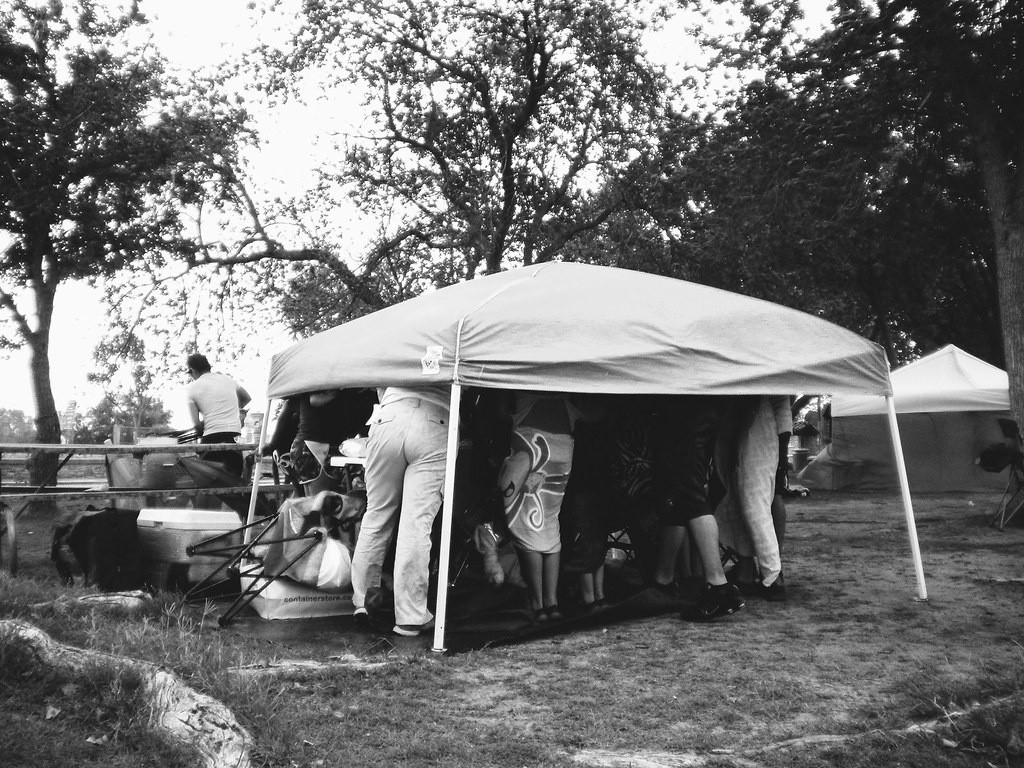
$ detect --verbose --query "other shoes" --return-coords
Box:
[353,606,434,636]
[753,578,784,594]
[567,597,609,622]
[643,570,675,593]
[517,605,561,629]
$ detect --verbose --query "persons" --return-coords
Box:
[246,383,792,635]
[187,354,251,514]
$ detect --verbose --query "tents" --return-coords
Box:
[240,261,929,653]
[831,344,1010,491]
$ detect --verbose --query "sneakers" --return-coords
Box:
[682,583,745,621]
